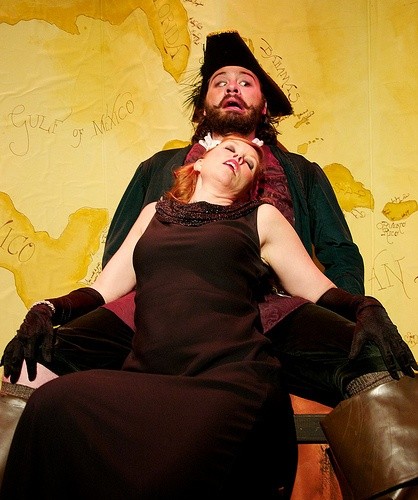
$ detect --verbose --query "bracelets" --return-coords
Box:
[26,300,56,314]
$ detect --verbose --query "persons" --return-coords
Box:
[101,30,365,300]
[0,136,417,499]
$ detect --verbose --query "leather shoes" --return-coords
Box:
[320,376,418,500]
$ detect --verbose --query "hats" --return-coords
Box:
[192,33,294,123]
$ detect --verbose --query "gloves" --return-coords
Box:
[0,287,105,385]
[315,288,418,380]
